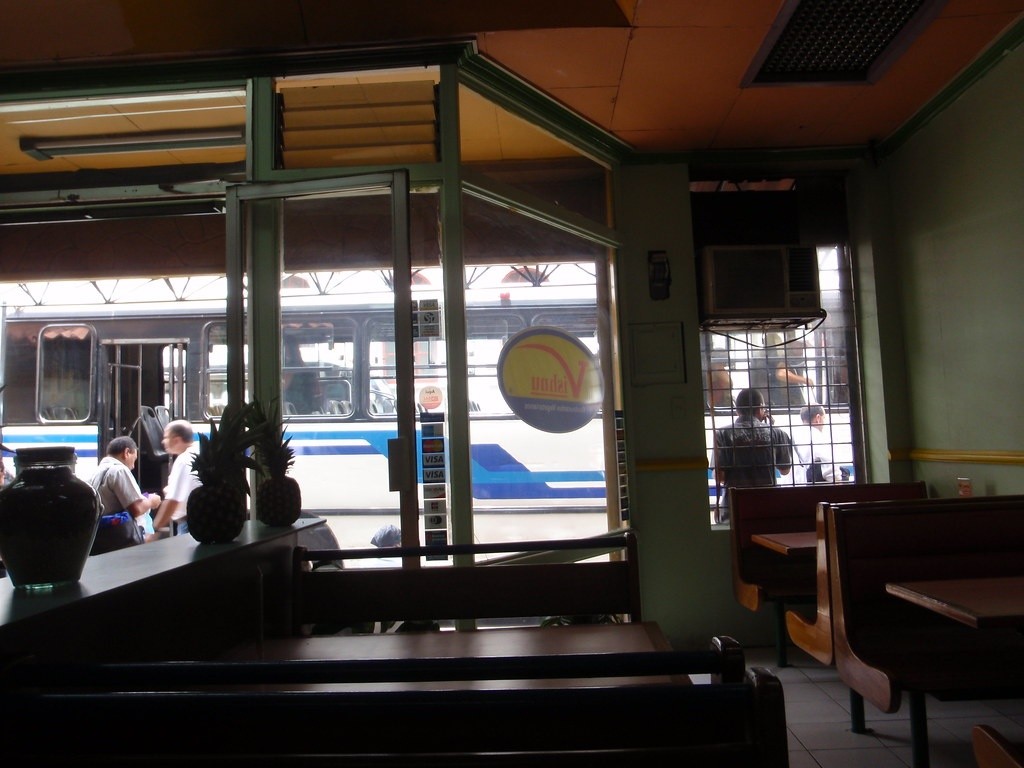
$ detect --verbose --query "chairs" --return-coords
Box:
[39,389,398,486]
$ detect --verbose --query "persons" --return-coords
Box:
[285,335,324,415]
[88,435,162,543]
[710,348,734,407]
[779,402,841,484]
[710,388,794,523]
[750,332,813,406]
[153,419,204,537]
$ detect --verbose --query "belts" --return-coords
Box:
[176,515,188,525]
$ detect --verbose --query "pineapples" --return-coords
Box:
[186,401,270,544]
[256,425,302,528]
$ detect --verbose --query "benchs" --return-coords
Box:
[286,530,648,628]
[40,636,788,767]
[727,479,1024,768]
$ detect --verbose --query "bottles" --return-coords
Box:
[0,445,105,590]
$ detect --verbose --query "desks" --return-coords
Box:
[179,619,691,693]
[884,575,1024,768]
[747,531,816,558]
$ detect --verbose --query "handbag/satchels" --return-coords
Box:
[90,509,145,556]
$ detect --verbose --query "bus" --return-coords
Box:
[2,284,855,557]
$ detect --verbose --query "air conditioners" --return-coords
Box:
[704,243,825,326]
